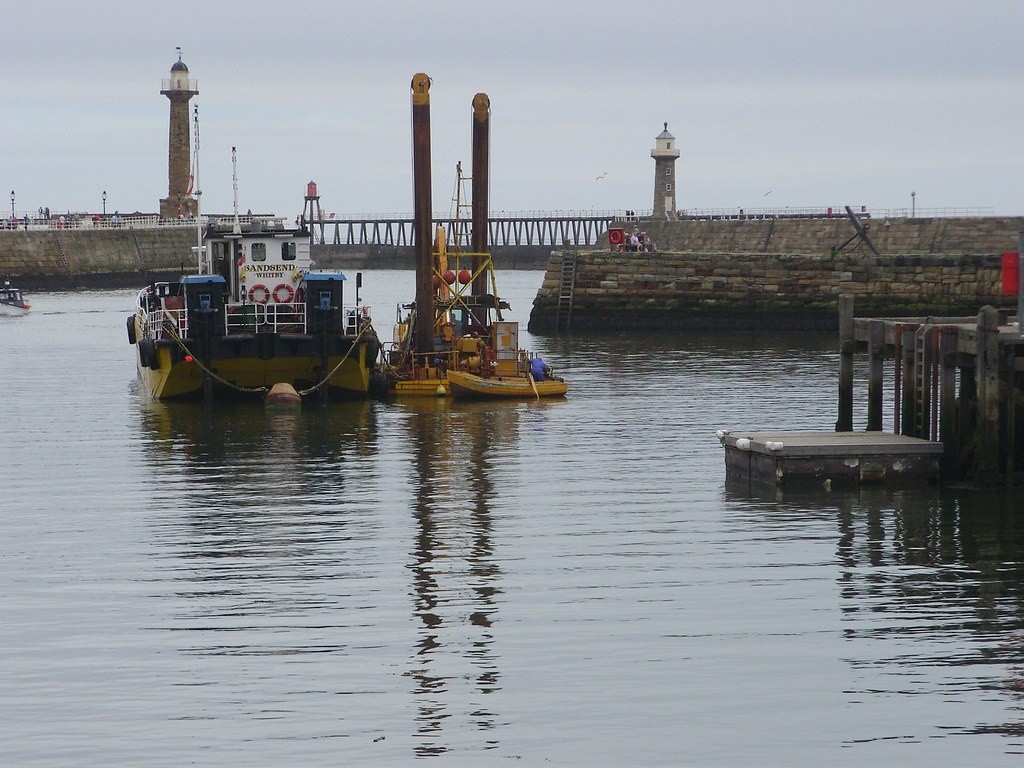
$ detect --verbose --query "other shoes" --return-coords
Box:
[654,249,658,252]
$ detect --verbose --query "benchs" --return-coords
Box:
[624,244,638,252]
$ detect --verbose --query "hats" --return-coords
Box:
[641,232,646,234]
[633,225,638,228]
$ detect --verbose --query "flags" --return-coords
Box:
[238,246,247,266]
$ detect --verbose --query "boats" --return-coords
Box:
[1,278,31,316]
[127,72,572,414]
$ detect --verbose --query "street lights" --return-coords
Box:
[10,190,16,218]
[102,190,107,222]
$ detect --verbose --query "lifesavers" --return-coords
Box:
[610,231,622,243]
[248,284,270,304]
[126,316,137,346]
[139,338,160,372]
[273,284,293,304]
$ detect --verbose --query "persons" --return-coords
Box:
[248,209,252,214]
[8,214,30,231]
[38,206,50,219]
[624,225,658,252]
[528,358,549,382]
[57,212,100,229]
[451,314,459,336]
[472,332,478,339]
